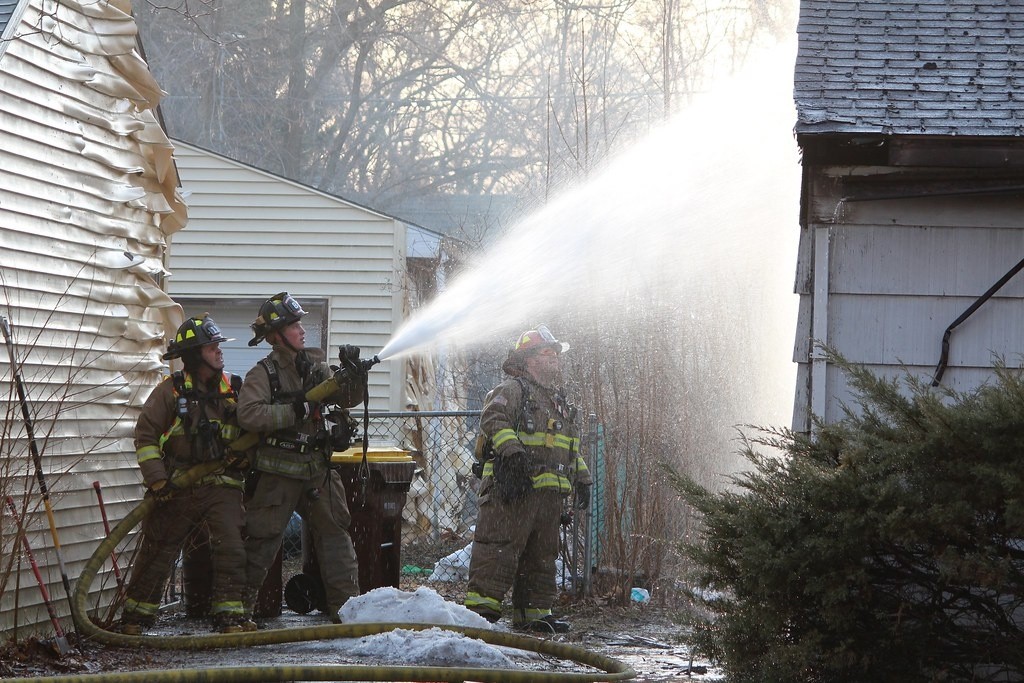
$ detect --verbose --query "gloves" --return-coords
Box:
[148,481,179,507]
[222,448,248,471]
[329,344,366,387]
[501,451,532,496]
[292,401,324,422]
[574,482,591,509]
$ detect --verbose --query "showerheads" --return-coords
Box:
[329,354,381,387]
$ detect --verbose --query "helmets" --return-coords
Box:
[162,312,228,360]
[247,292,310,346]
[502,323,563,376]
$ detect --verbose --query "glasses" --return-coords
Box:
[532,350,560,365]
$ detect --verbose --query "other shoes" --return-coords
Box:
[120,612,144,635]
[514,616,570,633]
[211,608,258,632]
[467,603,503,624]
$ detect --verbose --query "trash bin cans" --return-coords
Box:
[285,447,417,614]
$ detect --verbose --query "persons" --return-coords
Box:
[119,312,255,635]
[238,290,361,630]
[462,325,595,633]
